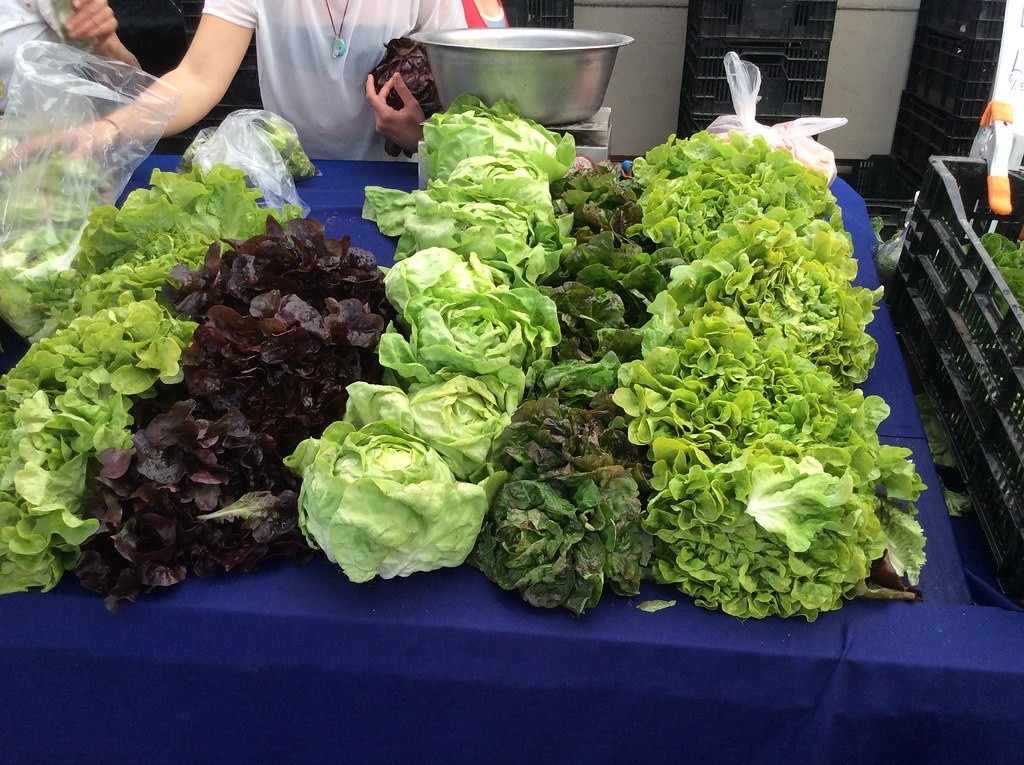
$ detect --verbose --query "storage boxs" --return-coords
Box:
[180,0,1024,597]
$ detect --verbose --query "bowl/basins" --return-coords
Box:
[410,27,635,125]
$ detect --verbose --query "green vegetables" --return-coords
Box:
[0,92,926,617]
[946,232,1024,419]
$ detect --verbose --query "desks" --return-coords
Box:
[0,155,1024,765]
